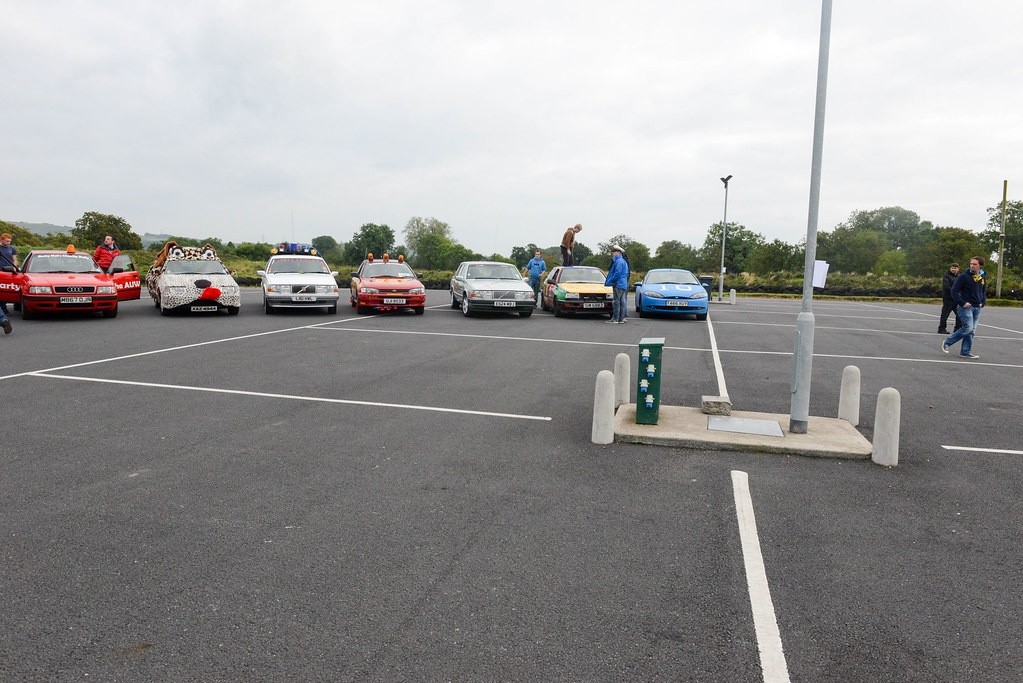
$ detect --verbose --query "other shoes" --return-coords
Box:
[2,318,12,334]
[937,331,950,334]
[604,319,619,324]
[619,319,624,324]
[959,352,979,359]
[942,340,950,354]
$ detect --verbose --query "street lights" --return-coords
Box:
[718,174,733,301]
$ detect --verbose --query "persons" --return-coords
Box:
[524,251,546,307]
[0,233,21,334]
[937,255,986,359]
[605,245,630,324]
[561,224,582,266]
[94,235,121,274]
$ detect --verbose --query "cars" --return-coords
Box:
[449,260,538,318]
[540,265,614,318]
[633,268,710,321]
[0,244,142,318]
[145,241,242,316]
[349,252,426,315]
[255,242,341,315]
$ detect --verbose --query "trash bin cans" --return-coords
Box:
[699,275,713,301]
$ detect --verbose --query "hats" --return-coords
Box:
[611,245,626,253]
[949,263,959,267]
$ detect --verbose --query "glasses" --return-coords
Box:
[951,267,957,269]
